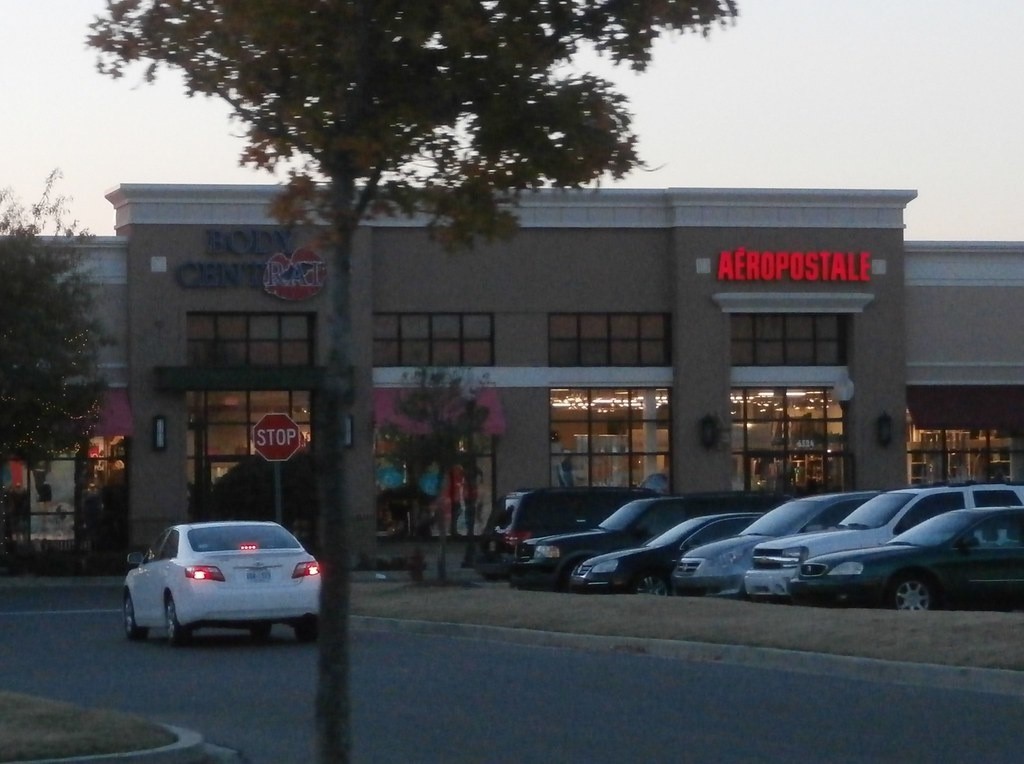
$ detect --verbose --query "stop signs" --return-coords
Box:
[253,412,301,464]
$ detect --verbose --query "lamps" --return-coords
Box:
[702,411,716,451]
[152,415,166,449]
[878,410,892,447]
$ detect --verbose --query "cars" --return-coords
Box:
[124,520,323,648]
[570,512,769,592]
[796,505,1024,610]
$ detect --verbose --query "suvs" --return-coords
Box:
[477,485,662,567]
[669,490,880,591]
[516,492,797,593]
[743,480,1024,599]
